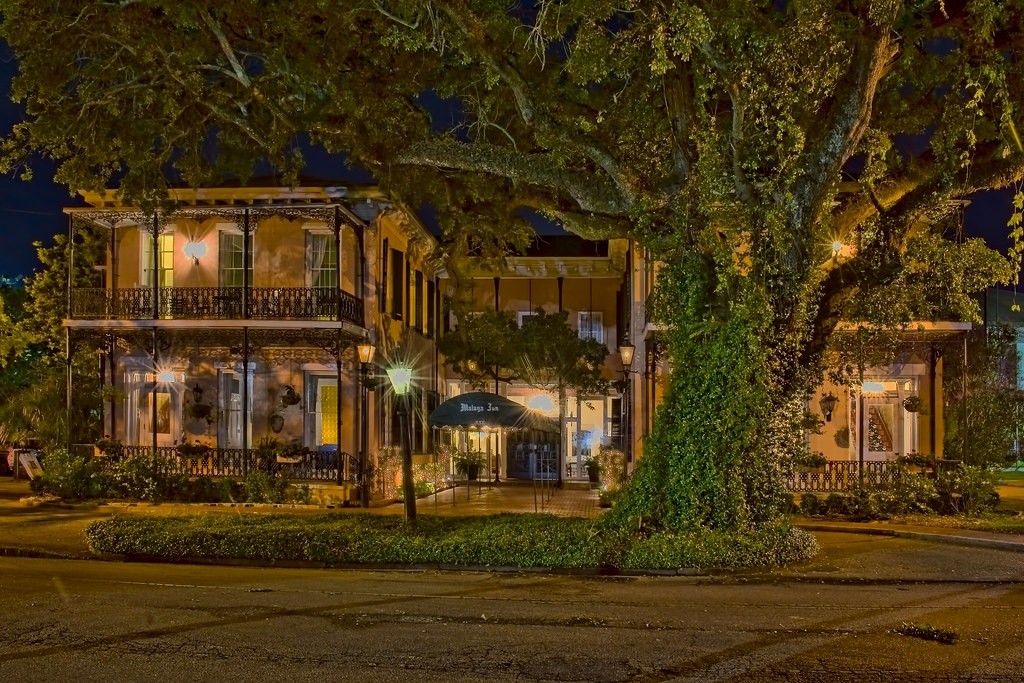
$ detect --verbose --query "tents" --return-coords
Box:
[429,391,561,512]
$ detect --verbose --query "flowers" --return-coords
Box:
[278,440,309,459]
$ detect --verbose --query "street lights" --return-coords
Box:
[356,329,378,510]
[855,325,888,497]
[615,327,641,484]
[385,357,418,523]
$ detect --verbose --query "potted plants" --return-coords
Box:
[582,456,605,482]
[902,395,924,412]
[280,384,301,406]
[361,377,379,391]
[452,447,488,480]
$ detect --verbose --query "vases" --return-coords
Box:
[276,453,305,463]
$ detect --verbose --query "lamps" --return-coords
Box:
[825,390,839,421]
[192,383,202,403]
